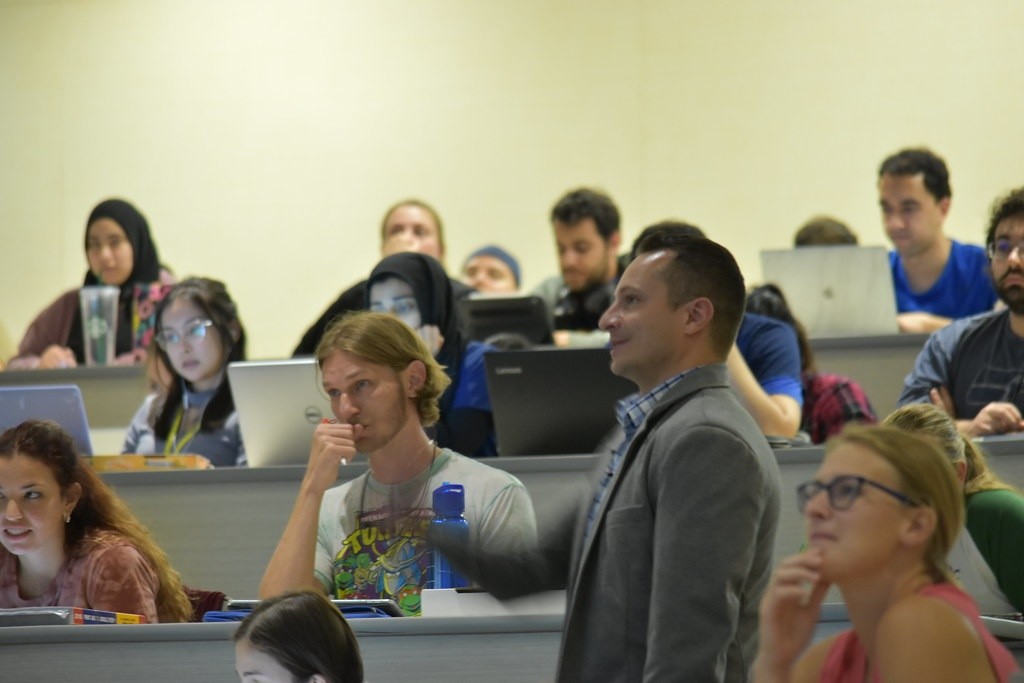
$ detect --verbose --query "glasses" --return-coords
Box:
[988,238,1024,260]
[795,475,924,516]
[154,319,214,352]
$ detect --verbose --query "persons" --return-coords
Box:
[0,421,194,624]
[745,285,877,446]
[795,217,858,246]
[257,313,538,614]
[6,198,177,366]
[883,404,1024,618]
[558,232,782,683]
[752,424,1021,683]
[531,187,642,329]
[231,590,364,683]
[902,184,1024,436]
[367,251,498,457]
[288,199,478,358]
[633,222,802,439]
[879,148,1004,334]
[452,245,519,295]
[123,275,253,467]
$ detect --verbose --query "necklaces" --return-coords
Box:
[358,441,436,527]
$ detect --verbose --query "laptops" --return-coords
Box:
[0,385,94,457]
[465,295,556,346]
[484,347,639,457]
[227,358,371,466]
[758,246,901,339]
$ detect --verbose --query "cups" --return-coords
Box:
[79,285,121,370]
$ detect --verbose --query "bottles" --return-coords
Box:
[428,482,472,589]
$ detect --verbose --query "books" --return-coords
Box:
[0,608,146,628]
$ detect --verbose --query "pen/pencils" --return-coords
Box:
[321,419,347,466]
